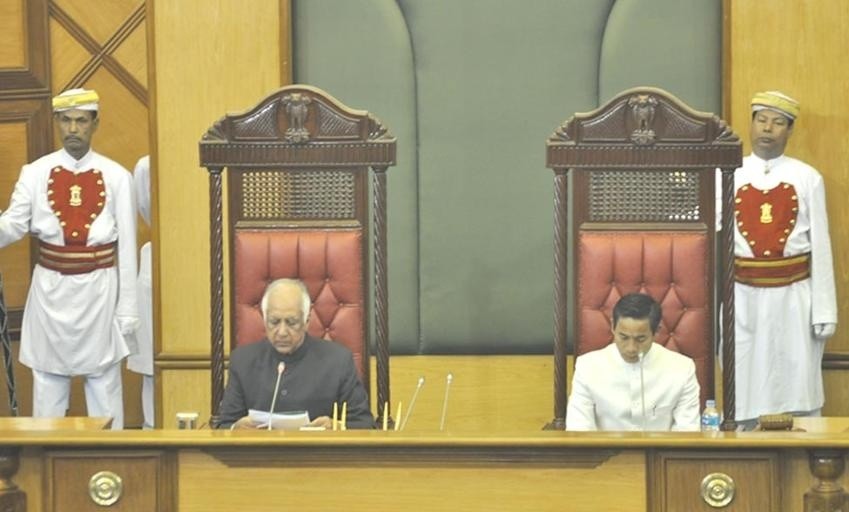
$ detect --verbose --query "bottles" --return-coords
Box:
[701,400,720,433]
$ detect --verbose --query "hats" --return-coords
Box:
[750,91,802,122]
[51,87,99,113]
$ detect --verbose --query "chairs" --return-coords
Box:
[546,86,743,429]
[200,86,397,431]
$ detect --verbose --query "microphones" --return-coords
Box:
[268,361,286,431]
[399,375,425,431]
[440,372,454,431]
[637,350,647,433]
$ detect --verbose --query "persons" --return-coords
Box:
[0,86,140,430]
[209,276,379,430]
[669,88,839,420]
[125,153,154,429]
[565,292,702,432]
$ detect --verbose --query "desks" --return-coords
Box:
[1,415,848,511]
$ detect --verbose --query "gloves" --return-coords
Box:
[119,315,143,334]
[814,323,837,339]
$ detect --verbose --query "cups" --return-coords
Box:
[176,413,199,429]
